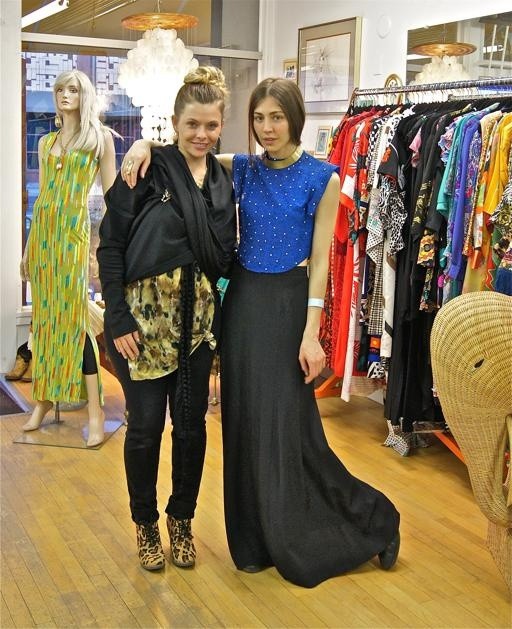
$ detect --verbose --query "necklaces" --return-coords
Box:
[264,148,297,162]
[186,160,207,189]
[56,128,81,170]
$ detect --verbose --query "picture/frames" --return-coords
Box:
[311,125,334,161]
[382,73,402,105]
[280,55,299,85]
[292,15,362,115]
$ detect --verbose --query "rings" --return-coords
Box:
[126,160,133,175]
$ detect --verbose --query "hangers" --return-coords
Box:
[343,74,511,122]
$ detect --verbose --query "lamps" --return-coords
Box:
[117,0,202,151]
[21,0,71,30]
[408,22,478,103]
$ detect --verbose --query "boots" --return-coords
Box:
[4,353,32,381]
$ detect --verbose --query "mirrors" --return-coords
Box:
[403,10,511,104]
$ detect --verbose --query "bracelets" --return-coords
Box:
[306,297,326,310]
[89,274,99,278]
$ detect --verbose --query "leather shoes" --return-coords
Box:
[378,529,400,570]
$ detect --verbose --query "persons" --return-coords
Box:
[95,65,237,572]
[120,76,402,592]
[21,68,118,448]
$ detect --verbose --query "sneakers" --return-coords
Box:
[166,514,197,567]
[135,519,165,570]
